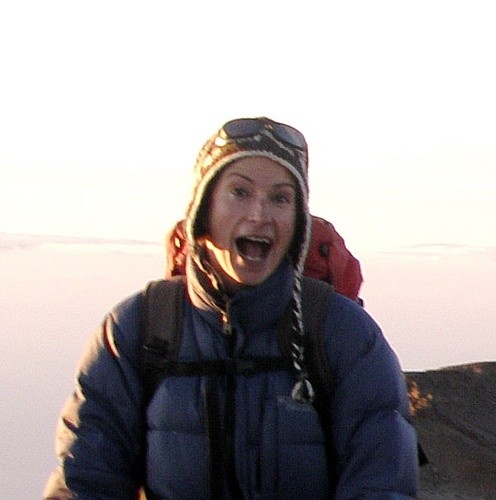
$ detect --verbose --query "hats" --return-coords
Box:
[183,117,317,404]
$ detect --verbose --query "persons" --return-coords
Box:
[42,117,423,500]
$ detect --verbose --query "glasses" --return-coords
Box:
[200,121,312,183]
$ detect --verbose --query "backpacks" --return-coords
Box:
[131,205,372,464]
